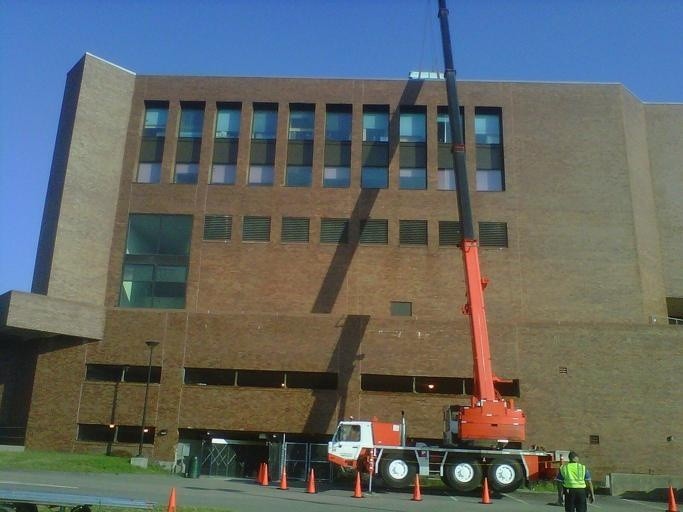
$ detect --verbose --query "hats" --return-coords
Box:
[568,452,577,459]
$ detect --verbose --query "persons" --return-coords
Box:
[555,450,595,511]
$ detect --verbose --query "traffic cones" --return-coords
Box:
[409,473,423,502]
[351,470,366,498]
[255,462,269,487]
[664,483,681,512]
[304,468,317,494]
[276,463,290,490]
[478,476,493,504]
[166,486,177,512]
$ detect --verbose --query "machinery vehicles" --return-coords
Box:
[326,2,565,496]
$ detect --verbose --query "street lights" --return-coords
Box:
[134,340,161,460]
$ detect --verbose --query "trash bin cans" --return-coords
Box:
[184,455,198,479]
